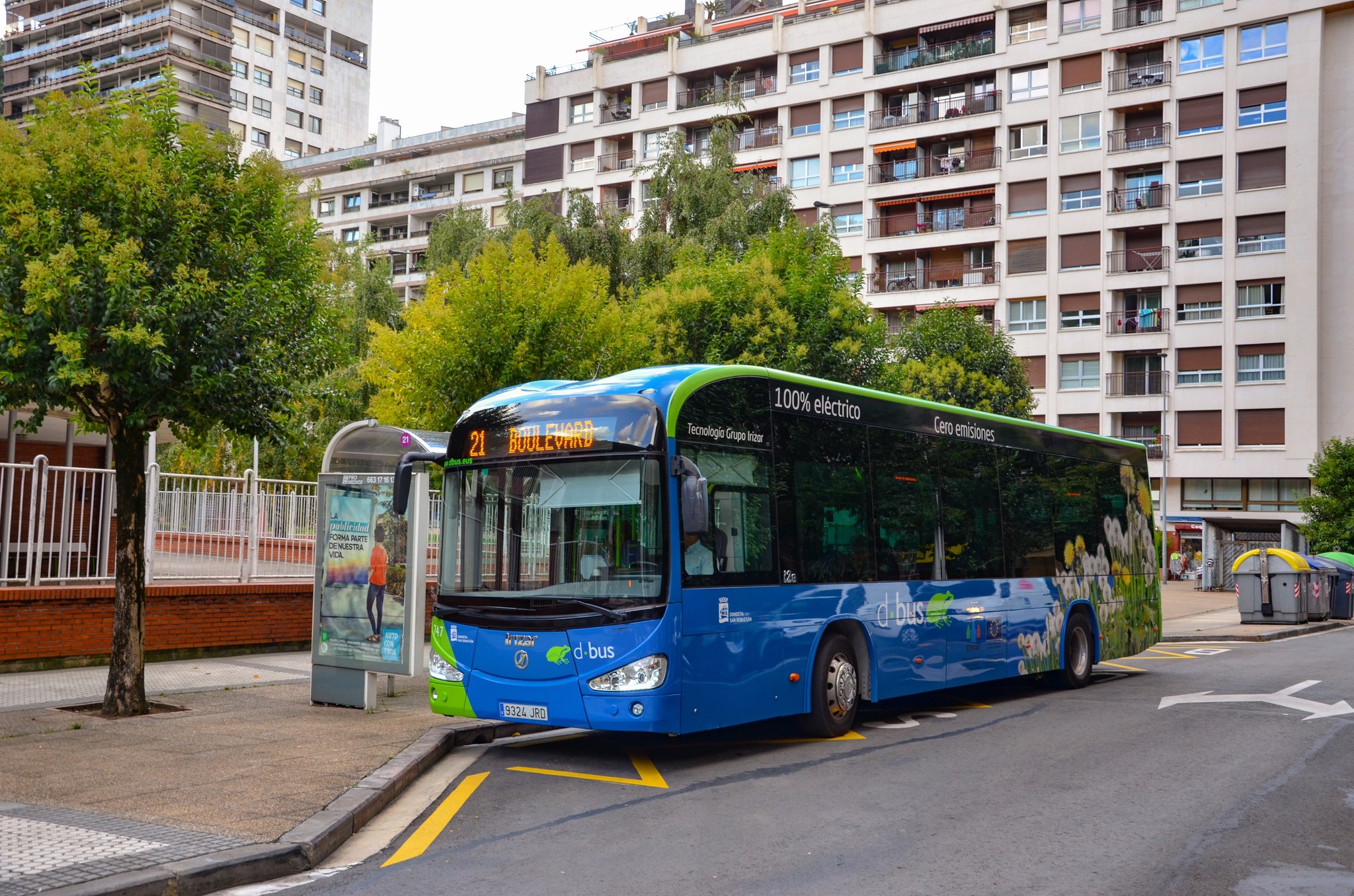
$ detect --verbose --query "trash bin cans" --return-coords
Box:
[1316,560,1340,622]
[1314,552,1354,620]
[1230,548,1312,625]
[1294,552,1331,623]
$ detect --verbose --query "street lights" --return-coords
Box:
[1157,352,1168,586]
[389,249,411,308]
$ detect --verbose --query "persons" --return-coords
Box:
[683,527,714,577]
[1186,546,1202,572]
[612,519,649,568]
[365,526,387,643]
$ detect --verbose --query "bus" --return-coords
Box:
[391,361,1161,740]
